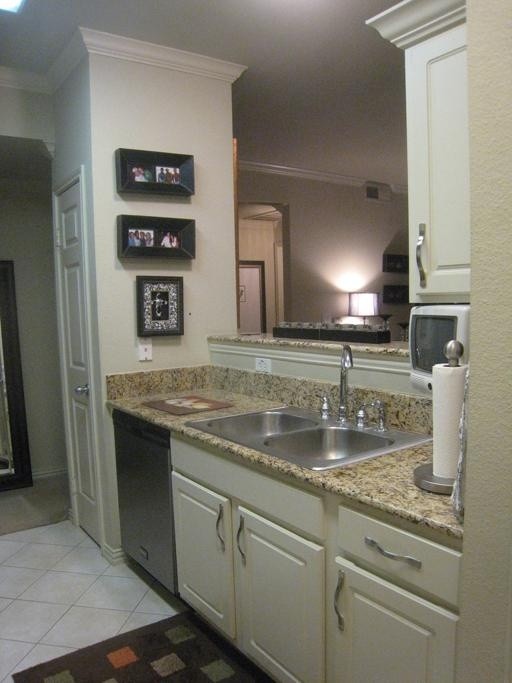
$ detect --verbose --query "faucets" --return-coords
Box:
[337,345,354,422]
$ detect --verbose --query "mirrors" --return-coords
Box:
[0,258,37,492]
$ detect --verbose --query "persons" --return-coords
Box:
[130,165,180,184]
[129,228,180,250]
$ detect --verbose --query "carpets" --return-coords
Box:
[10,605,275,681]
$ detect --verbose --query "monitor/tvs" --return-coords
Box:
[409,304,470,397]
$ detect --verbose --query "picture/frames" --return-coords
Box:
[383,283,409,303]
[114,145,196,198]
[133,272,186,339]
[115,212,197,263]
[382,253,408,273]
[237,258,269,334]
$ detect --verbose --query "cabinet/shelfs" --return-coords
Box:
[169,431,326,683]
[325,489,460,680]
[407,17,470,298]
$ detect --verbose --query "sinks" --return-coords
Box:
[186,406,319,466]
[262,426,432,492]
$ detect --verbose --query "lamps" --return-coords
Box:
[347,290,382,326]
